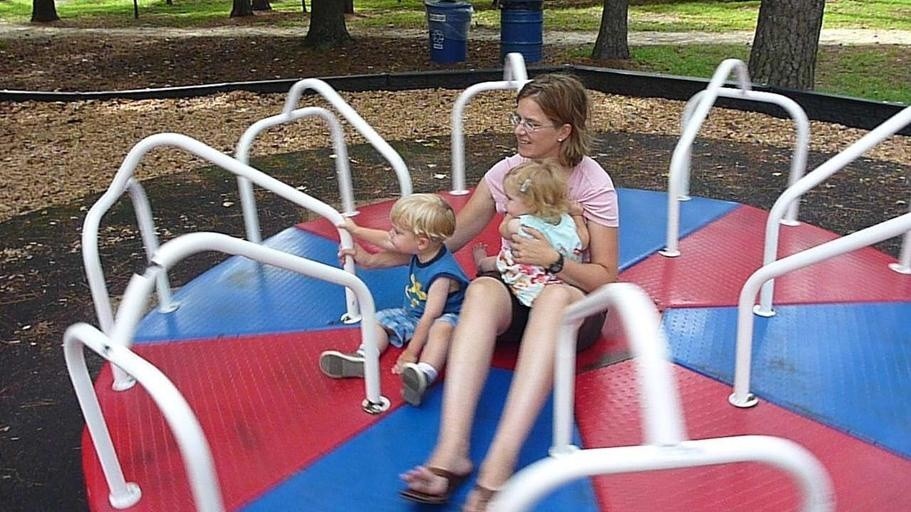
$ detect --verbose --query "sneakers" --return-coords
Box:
[400,361,428,408]
[318,349,364,380]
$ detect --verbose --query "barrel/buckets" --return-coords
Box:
[500,8,543,65]
[426,2,472,62]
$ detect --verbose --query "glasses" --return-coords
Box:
[509,112,558,134]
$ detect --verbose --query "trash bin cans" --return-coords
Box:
[424,2,544,64]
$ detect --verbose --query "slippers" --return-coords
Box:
[399,465,469,504]
[463,483,499,512]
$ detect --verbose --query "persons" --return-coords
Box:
[471,160,592,311]
[320,193,473,409]
[339,73,622,512]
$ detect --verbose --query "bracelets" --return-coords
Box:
[548,253,565,275]
